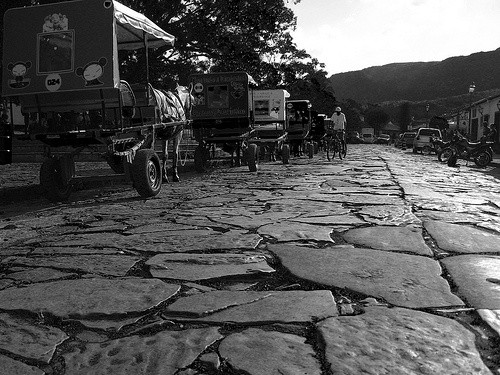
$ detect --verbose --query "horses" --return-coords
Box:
[154,82,194,184]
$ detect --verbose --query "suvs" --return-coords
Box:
[376,133,391,146]
[362,133,374,144]
[413,127,444,154]
[394,133,402,147]
[345,130,360,144]
[401,132,417,147]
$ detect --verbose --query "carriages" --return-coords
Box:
[1,1,198,198]
[188,70,332,172]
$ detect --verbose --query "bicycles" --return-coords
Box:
[325,127,348,161]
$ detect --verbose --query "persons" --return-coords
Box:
[330,107,346,152]
[461,121,468,136]
[482,121,497,161]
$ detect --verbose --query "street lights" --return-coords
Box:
[426,103,429,128]
[468,81,476,133]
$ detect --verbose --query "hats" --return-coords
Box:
[335,107,341,112]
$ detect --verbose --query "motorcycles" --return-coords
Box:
[437,131,496,167]
[421,134,444,156]
